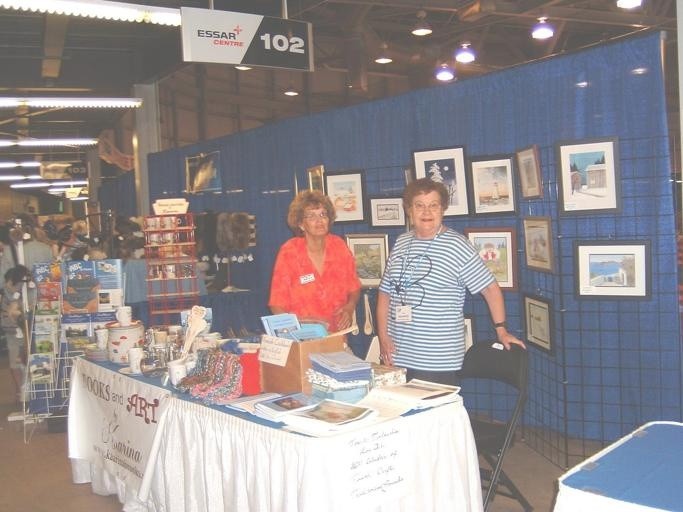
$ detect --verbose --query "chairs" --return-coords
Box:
[456,338,535,512]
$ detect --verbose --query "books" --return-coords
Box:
[254,392,325,420]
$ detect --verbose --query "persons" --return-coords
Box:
[492,322,506,329]
[57,225,72,247]
[43,223,58,258]
[269,191,361,343]
[58,221,86,260]
[376,179,527,386]
[0,216,51,410]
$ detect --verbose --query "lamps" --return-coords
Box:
[455,43,475,63]
[531,18,554,39]
[435,64,455,81]
[374,44,393,64]
[284,81,300,97]
[410,11,433,36]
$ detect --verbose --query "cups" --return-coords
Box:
[115,306,132,326]
[168,325,182,334]
[95,328,109,350]
[128,348,143,372]
[155,331,167,345]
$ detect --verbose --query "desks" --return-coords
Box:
[68,349,484,512]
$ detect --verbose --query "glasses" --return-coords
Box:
[302,211,328,219]
[414,200,440,210]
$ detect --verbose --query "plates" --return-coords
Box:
[119,365,154,375]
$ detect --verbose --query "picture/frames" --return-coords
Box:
[572,240,652,301]
[522,216,555,275]
[367,194,407,229]
[411,145,472,219]
[323,169,368,224]
[523,292,556,357]
[516,144,544,199]
[469,153,519,217]
[343,232,390,290]
[465,313,476,354]
[463,227,519,293]
[553,135,623,217]
[185,151,223,193]
[308,165,325,196]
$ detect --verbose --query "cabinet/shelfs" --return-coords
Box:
[142,212,200,328]
[23,300,86,444]
